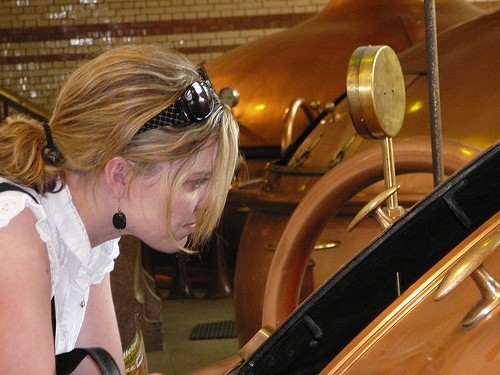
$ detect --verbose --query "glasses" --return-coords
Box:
[134,66,214,135]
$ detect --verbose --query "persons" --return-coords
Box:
[0,44,241,374]
